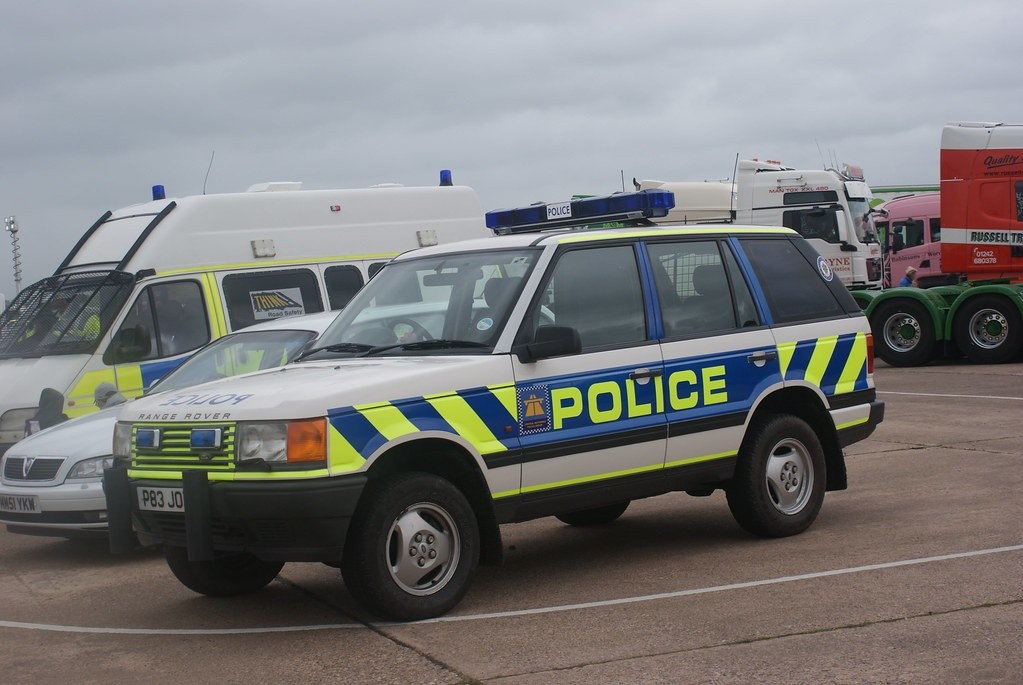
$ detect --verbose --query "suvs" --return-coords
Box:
[98,186,888,619]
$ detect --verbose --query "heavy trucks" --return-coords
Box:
[621,119,1023,370]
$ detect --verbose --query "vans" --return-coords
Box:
[0,168,503,462]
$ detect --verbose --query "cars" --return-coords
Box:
[0,299,555,539]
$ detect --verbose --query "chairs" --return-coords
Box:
[174,299,211,351]
[684,262,748,333]
[461,277,534,353]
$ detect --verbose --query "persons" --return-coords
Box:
[0,284,181,348]
[24,388,71,437]
[93,382,127,410]
